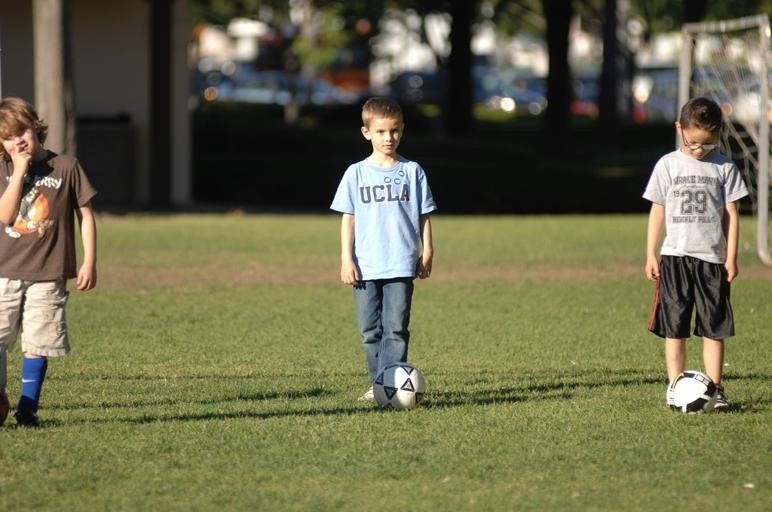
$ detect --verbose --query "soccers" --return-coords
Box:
[667,369,717,416]
[373,362,425,411]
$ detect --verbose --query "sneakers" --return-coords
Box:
[711,382,730,411]
[357,385,375,401]
[13,408,42,429]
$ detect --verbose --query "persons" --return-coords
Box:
[638,96,750,415]
[0,96,100,432]
[325,95,435,405]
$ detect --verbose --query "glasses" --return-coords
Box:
[681,127,720,152]
